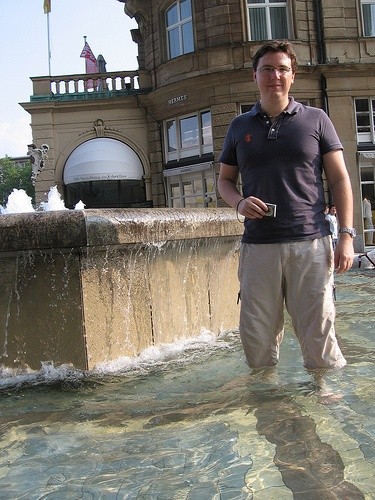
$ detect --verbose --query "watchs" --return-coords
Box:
[339,227,356,238]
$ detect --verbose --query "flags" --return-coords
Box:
[80,42,102,89]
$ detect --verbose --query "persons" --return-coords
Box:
[218,38,356,371]
[325,192,373,252]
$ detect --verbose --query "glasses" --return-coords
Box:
[256,67,293,74]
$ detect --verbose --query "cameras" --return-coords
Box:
[264,203,276,217]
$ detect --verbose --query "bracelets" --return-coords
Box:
[236,199,250,223]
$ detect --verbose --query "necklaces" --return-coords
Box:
[267,115,279,118]
